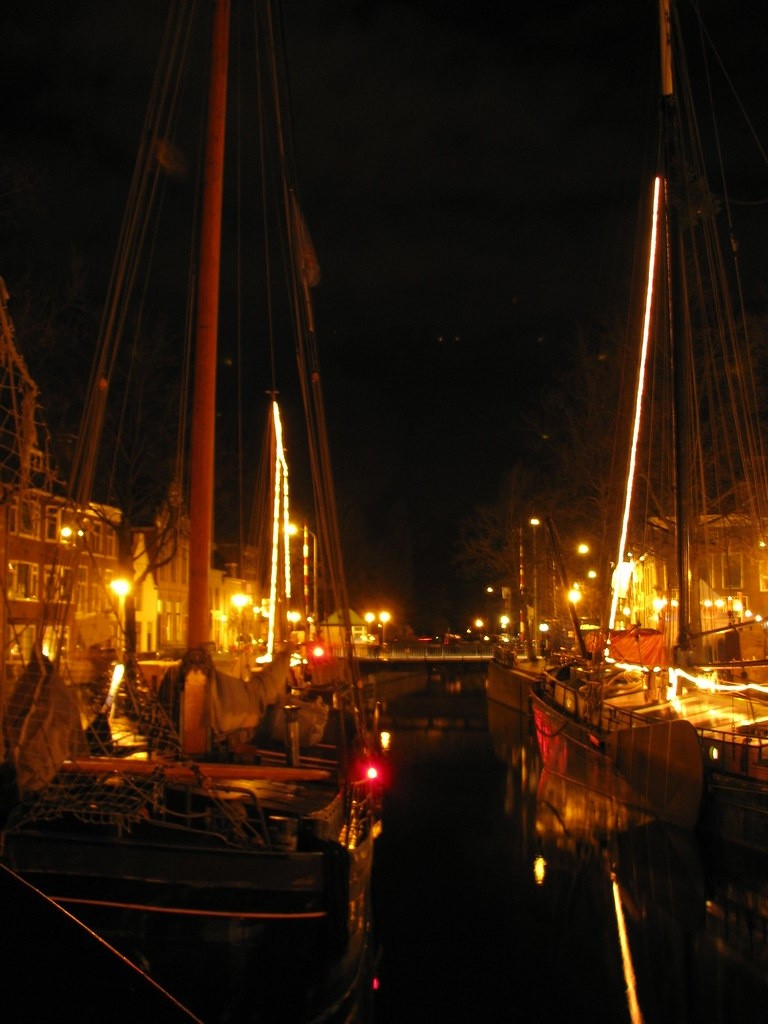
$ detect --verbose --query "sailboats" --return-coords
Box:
[0,0,768,1024]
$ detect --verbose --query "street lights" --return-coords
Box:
[285,524,317,639]
[530,508,538,648]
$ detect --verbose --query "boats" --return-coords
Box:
[488,511,588,714]
